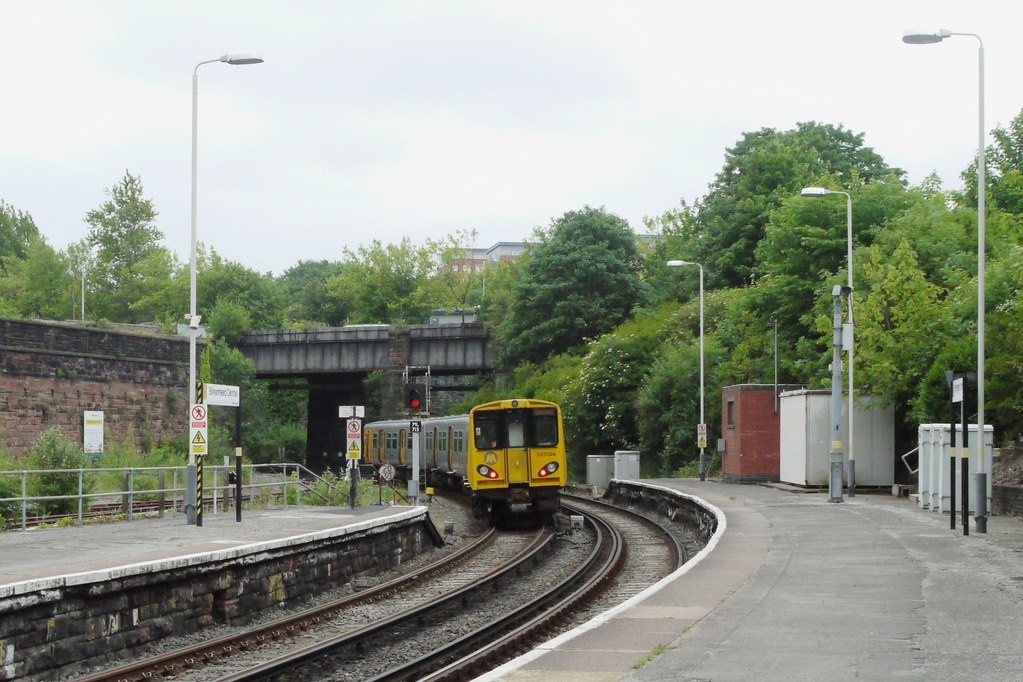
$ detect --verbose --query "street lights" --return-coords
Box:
[671,253,714,494]
[906,18,1002,535]
[178,46,275,523]
[805,182,867,497]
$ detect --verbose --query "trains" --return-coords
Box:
[359,398,563,531]
